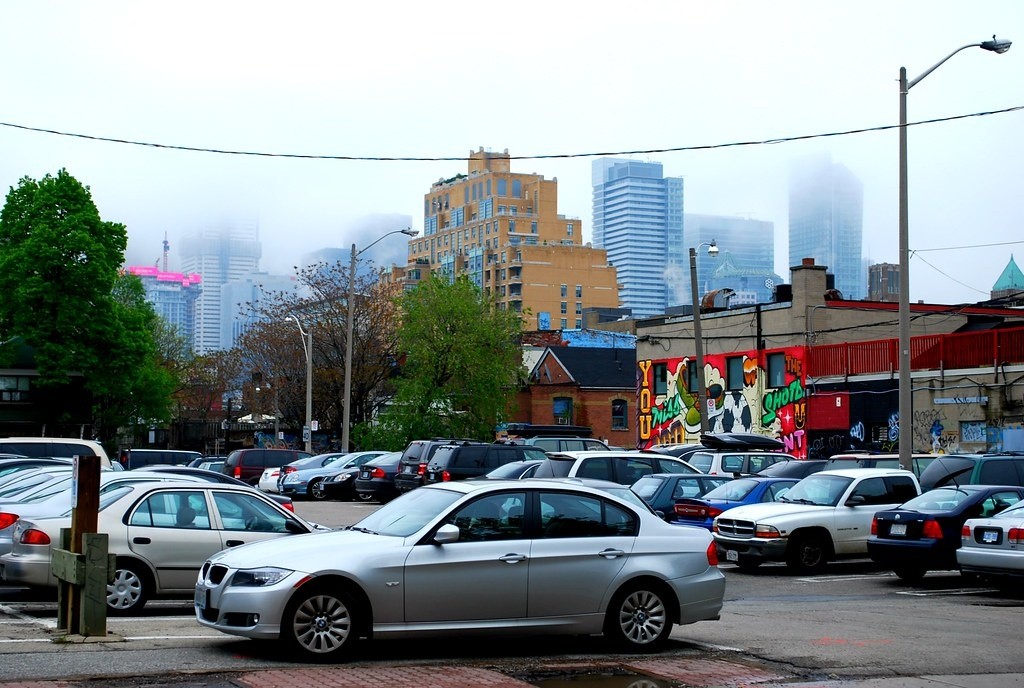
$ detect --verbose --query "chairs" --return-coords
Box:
[623,466,638,484]
[174,507,196,528]
[742,460,755,472]
[505,506,522,526]
[469,503,502,537]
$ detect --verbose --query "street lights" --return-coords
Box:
[340,228,420,453]
[895,34,1016,469]
[285,314,314,453]
[686,238,720,437]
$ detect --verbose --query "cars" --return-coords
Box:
[671,476,829,534]
[0,428,828,567]
[0,481,329,616]
[955,498,1024,597]
[866,484,1024,578]
[710,467,923,574]
[191,477,728,664]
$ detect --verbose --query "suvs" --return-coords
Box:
[824,448,951,483]
[920,450,1024,499]
